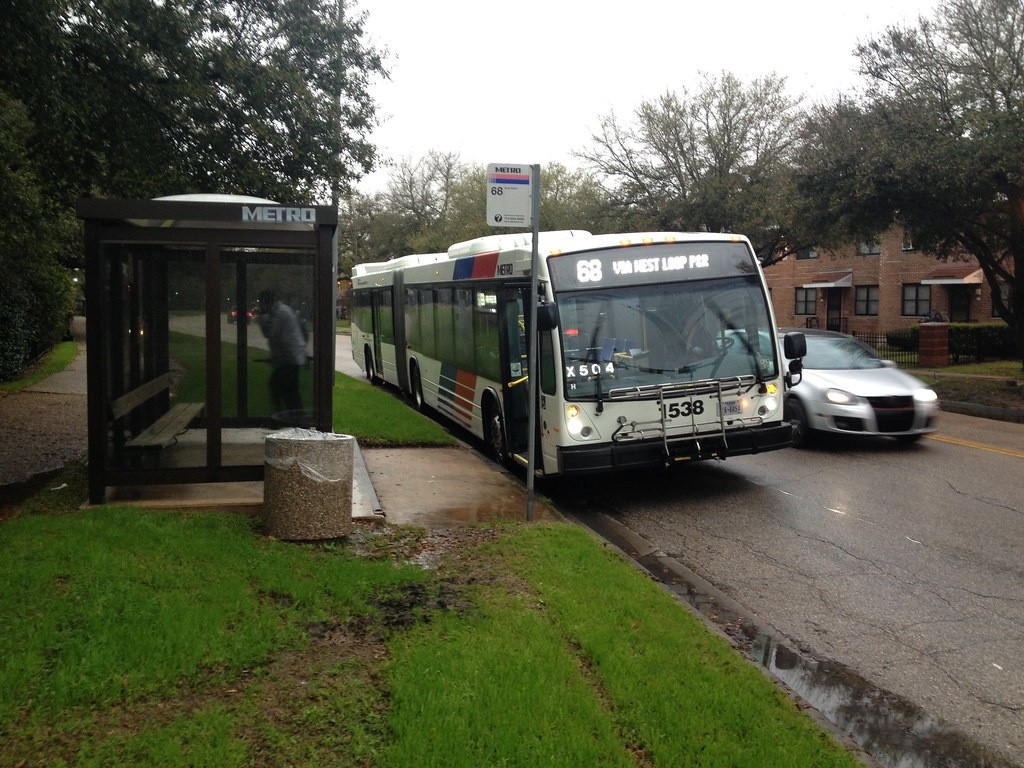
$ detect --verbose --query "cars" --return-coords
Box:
[227,304,255,326]
[711,326,940,443]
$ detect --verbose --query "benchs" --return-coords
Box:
[110,369,206,471]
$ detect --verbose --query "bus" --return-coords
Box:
[349,229,807,498]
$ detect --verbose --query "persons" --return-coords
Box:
[255,290,306,412]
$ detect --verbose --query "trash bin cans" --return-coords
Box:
[261,431,355,540]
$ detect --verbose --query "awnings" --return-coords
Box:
[794,270,852,288]
[921,268,983,284]
